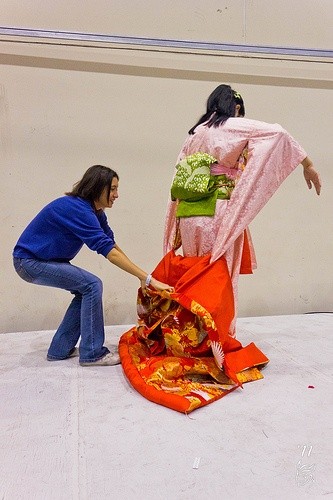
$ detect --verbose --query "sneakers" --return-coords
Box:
[47,347,81,361]
[80,351,122,366]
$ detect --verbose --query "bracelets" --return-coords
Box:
[144,274,152,287]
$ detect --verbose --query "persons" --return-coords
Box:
[10,164,176,367]
[163,83,321,341]
